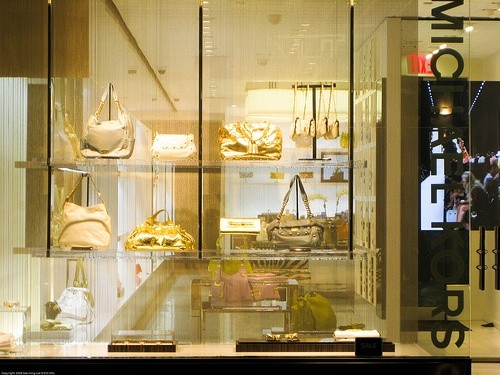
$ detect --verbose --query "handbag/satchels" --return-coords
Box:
[151,133,196,159]
[325,120,339,139]
[306,119,315,138]
[125,209,195,250]
[266,175,323,250]
[58,173,111,249]
[292,290,337,331]
[211,260,253,306]
[80,86,134,159]
[58,259,90,321]
[316,119,328,138]
[218,122,282,161]
[291,118,301,141]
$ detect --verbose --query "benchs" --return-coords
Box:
[191,279,299,344]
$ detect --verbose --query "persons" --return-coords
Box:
[446,136,500,229]
